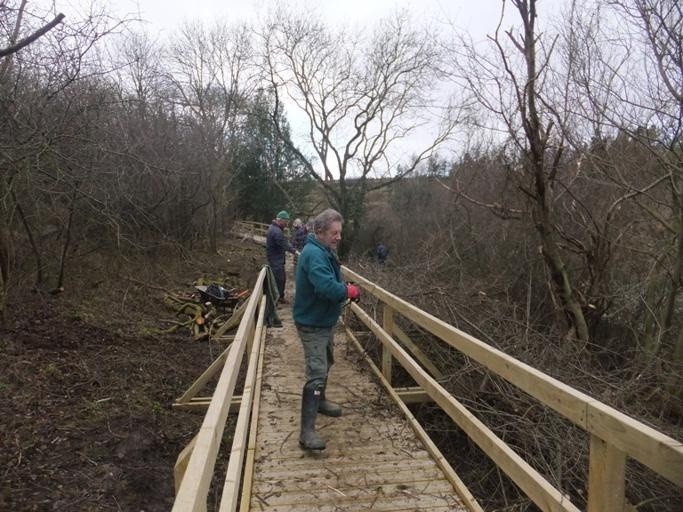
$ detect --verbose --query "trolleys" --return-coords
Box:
[195,285,249,321]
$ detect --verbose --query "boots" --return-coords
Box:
[299,388,327,450]
[318,375,341,417]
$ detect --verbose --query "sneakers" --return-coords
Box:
[281,299,289,304]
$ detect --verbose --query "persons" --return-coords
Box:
[292,218,316,267]
[374,241,388,264]
[293,208,361,450]
[266,210,301,304]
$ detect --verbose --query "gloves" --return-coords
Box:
[347,285,360,298]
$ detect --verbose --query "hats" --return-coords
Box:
[276,211,289,219]
